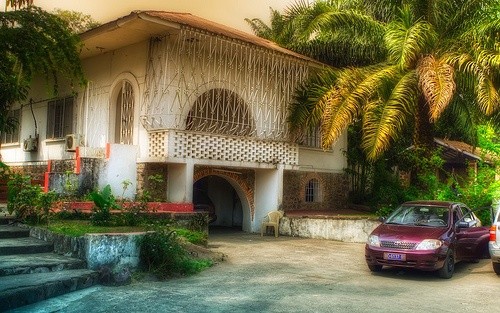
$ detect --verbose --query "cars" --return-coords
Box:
[366,199,495,278]
[489,206,500,275]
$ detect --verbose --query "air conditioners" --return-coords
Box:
[23,138,37,152]
[63,132,84,154]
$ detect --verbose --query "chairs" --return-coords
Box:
[440,211,457,225]
[260,210,284,239]
[409,209,430,223]
